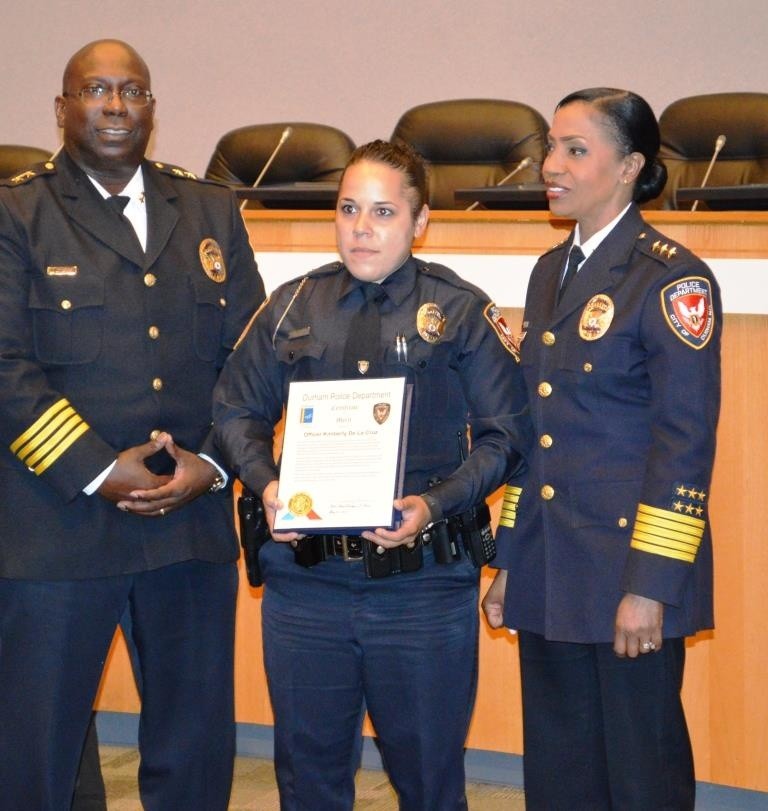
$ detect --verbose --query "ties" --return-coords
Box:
[559,245,585,293]
[343,280,388,380]
[105,195,146,260]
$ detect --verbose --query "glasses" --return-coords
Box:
[64,85,153,108]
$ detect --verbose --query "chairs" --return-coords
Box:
[390,99,550,210]
[205,122,357,209]
[0,145,54,179]
[638,92,768,211]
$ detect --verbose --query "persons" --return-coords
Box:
[0,38,267,810]
[481,88,722,811]
[210,139,534,811]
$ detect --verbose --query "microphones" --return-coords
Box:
[691,135,726,212]
[240,127,292,210]
[466,156,532,210]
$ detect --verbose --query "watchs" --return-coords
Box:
[209,476,223,492]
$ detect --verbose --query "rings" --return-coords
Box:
[160,509,165,516]
[642,640,656,650]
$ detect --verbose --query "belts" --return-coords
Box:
[304,514,469,562]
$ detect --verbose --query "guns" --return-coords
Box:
[238,484,267,589]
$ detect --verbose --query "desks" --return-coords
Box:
[91,209,768,797]
